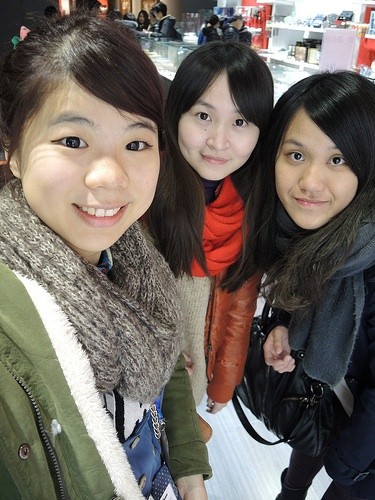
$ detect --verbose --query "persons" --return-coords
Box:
[42,0,253,50]
[136,41,275,442]
[220,70,375,500]
[0,10,213,500]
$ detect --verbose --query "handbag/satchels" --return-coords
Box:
[230,285,342,460]
